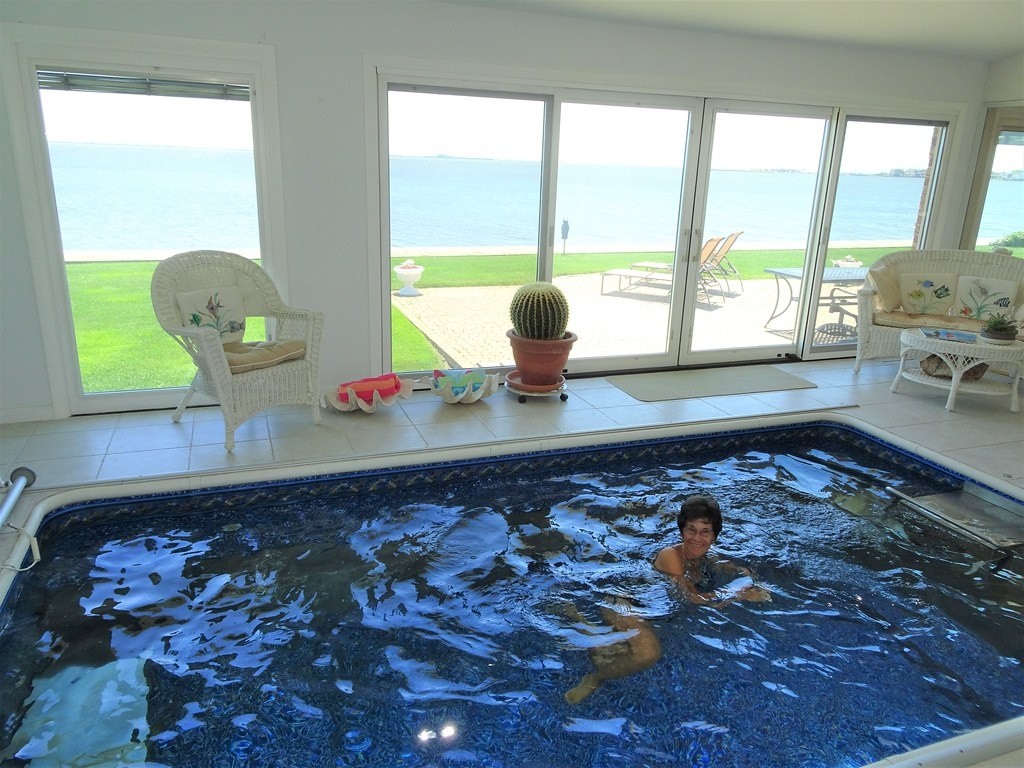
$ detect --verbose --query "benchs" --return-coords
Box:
[850,249,1023,375]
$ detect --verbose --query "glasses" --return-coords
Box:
[684,525,715,537]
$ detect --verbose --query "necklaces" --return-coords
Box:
[682,543,696,568]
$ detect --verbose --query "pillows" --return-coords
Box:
[868,262,903,314]
[898,272,958,316]
[954,275,1019,324]
[176,284,246,345]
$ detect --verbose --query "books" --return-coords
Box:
[939,330,976,343]
[919,328,941,338]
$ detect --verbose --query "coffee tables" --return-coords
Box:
[888,326,1023,414]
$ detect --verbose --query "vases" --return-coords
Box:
[393,265,424,297]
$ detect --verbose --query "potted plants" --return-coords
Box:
[504,281,578,395]
[979,313,1020,345]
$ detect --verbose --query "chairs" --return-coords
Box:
[628,230,744,298]
[150,250,326,452]
[598,236,727,312]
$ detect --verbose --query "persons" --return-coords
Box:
[552,494,773,706]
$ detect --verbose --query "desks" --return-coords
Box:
[763,266,869,348]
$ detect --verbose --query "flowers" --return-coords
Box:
[401,259,416,268]
